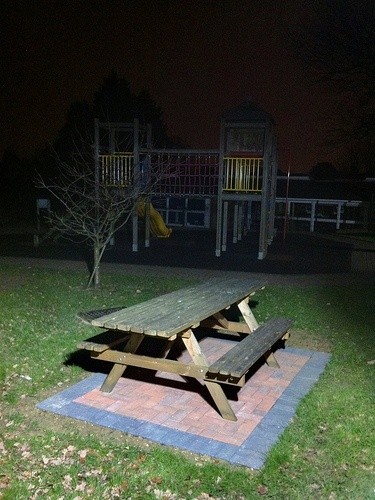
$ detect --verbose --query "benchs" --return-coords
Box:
[78,324,132,363]
[216,317,293,387]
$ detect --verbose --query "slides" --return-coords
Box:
[135,198,172,239]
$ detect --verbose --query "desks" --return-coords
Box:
[87,276,284,420]
